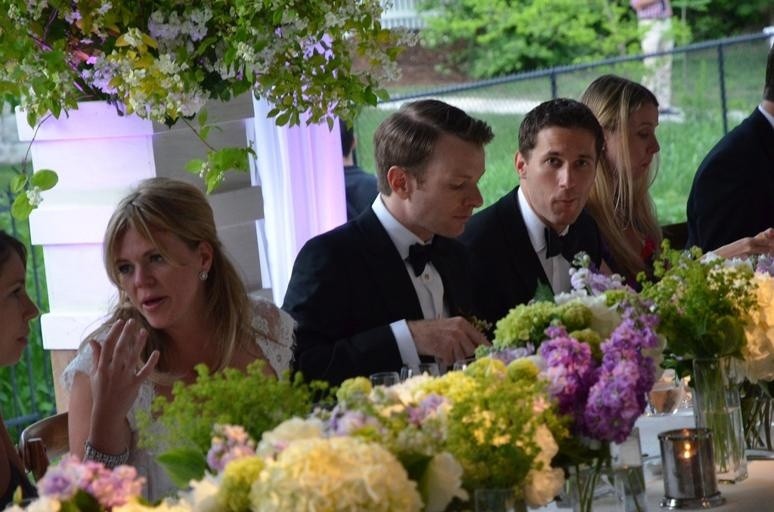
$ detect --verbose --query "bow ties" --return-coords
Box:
[543,225,584,259]
[405,239,447,277]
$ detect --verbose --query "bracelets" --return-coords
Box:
[84,441,128,469]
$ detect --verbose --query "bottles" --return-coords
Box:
[691,356,749,486]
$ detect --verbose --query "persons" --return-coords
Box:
[281,98,497,406]
[337,114,377,224]
[0,228,42,511]
[455,97,638,345]
[58,175,302,508]
[580,73,773,294]
[627,0,681,116]
[682,45,772,259]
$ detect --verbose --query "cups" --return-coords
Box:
[646,368,686,417]
[368,357,477,388]
